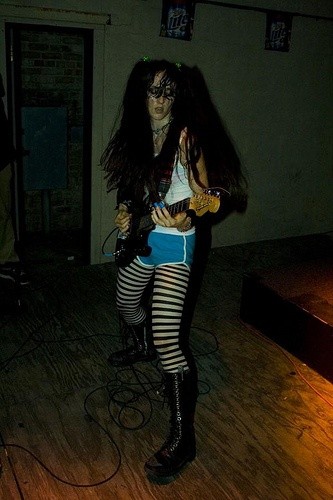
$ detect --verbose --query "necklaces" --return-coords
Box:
[152,120,171,136]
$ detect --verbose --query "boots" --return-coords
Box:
[106,316,156,367]
[143,363,198,477]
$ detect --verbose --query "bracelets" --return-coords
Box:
[180,217,191,230]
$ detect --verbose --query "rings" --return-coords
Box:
[161,223,165,227]
[165,221,170,224]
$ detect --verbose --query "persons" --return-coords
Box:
[99,60,242,476]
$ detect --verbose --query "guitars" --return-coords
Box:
[114,188,220,269]
